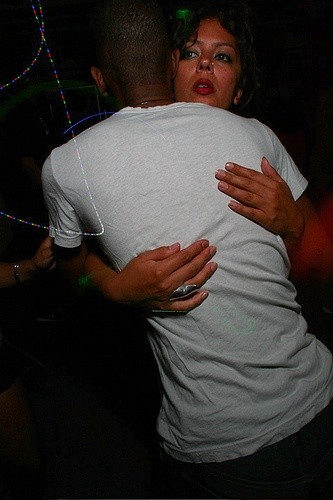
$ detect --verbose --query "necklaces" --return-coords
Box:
[135,98,176,109]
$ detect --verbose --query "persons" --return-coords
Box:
[79,0,306,499]
[1,229,68,499]
[39,0,333,499]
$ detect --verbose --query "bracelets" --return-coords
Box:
[13,259,26,287]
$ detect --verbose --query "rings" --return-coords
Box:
[167,283,198,302]
